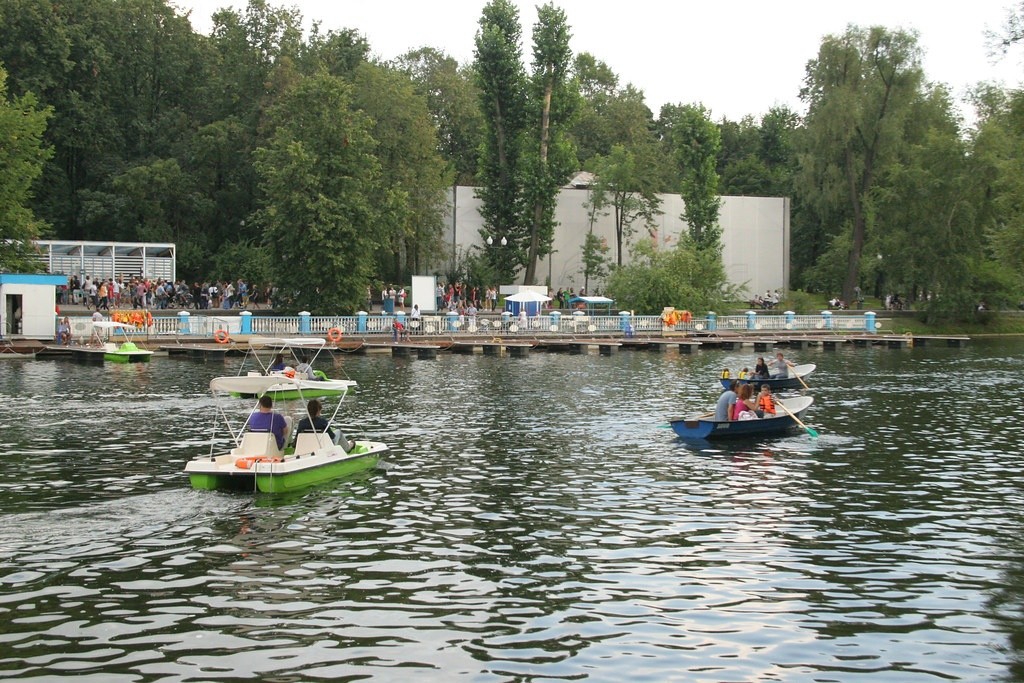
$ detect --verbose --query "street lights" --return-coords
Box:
[487,236,508,300]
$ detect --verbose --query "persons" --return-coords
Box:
[509,309,527,321]
[410,304,421,334]
[393,318,411,342]
[381,285,405,310]
[55,272,279,310]
[750,289,780,309]
[60,317,72,345]
[366,284,373,311]
[714,380,780,420]
[87,309,103,345]
[830,297,849,310]
[721,353,795,379]
[294,399,355,454]
[435,280,498,311]
[549,286,587,309]
[458,303,477,334]
[268,353,325,381]
[249,395,293,453]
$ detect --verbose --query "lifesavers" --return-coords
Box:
[236,457,282,469]
[328,328,342,341]
[147,312,152,327]
[215,330,229,343]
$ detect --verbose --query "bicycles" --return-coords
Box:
[168,296,197,310]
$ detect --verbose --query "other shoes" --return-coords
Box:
[347,439,356,454]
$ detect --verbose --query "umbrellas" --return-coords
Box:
[503,290,552,302]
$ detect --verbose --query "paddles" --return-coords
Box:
[697,412,715,418]
[786,363,809,389]
[776,400,819,437]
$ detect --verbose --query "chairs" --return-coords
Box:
[236,429,285,458]
[295,424,348,456]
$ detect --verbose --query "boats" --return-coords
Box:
[92,321,154,362]
[210,338,359,399]
[184,376,390,492]
[667,397,814,439]
[719,363,816,390]
